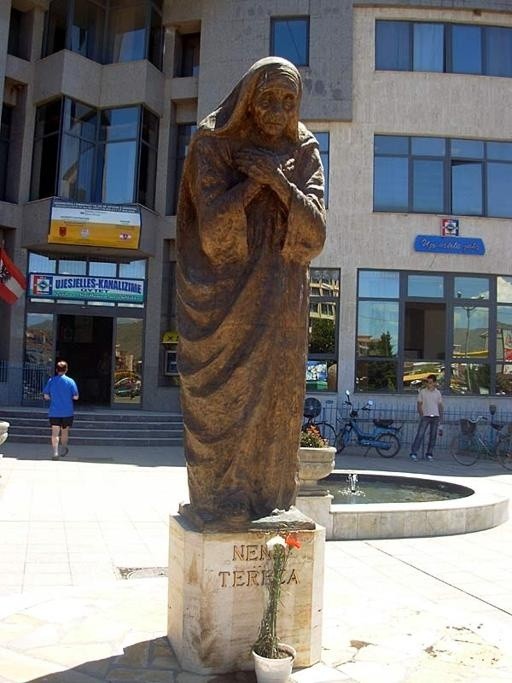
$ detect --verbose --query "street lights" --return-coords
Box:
[457,291,483,358]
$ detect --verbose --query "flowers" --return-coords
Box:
[299,425,327,447]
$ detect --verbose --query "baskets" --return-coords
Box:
[461,418,476,433]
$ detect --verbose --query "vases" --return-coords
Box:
[297,447,336,484]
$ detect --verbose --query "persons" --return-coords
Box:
[409,374,445,462]
[130,375,141,400]
[171,53,329,524]
[42,360,80,460]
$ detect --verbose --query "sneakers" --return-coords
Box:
[409,453,434,463]
[53,448,69,460]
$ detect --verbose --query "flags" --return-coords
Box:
[0,247,28,306]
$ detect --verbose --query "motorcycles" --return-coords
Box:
[334,389,406,459]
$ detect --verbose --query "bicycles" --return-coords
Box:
[450,415,512,473]
[302,404,338,449]
